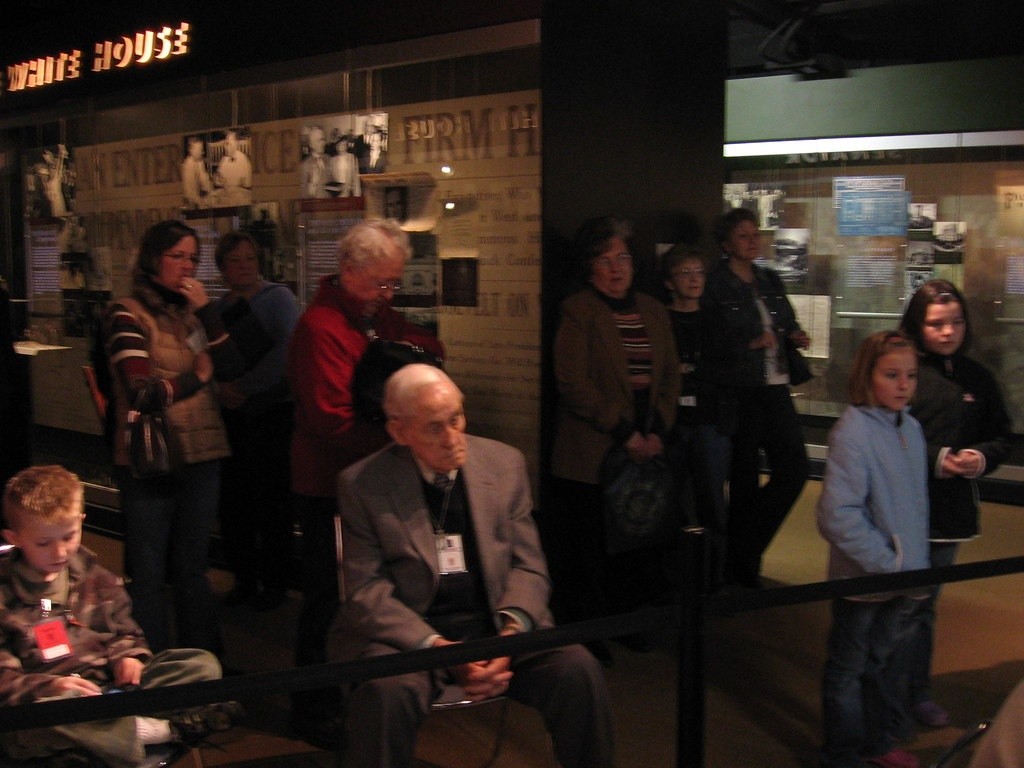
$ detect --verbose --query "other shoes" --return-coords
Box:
[25,745,104,767]
[291,710,342,750]
[255,591,282,612]
[157,700,242,750]
[614,632,652,652]
[914,700,950,728]
[587,641,612,665]
[866,745,920,767]
[223,583,251,606]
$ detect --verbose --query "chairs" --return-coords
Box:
[333,509,512,758]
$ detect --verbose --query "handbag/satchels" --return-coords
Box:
[599,446,684,550]
[130,378,182,498]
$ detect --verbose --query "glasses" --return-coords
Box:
[672,268,707,278]
[591,255,633,268]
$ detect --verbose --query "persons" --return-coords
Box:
[386,189,405,219]
[332,363,616,768]
[57,217,94,290]
[0,462,224,768]
[550,213,683,669]
[704,207,814,611]
[183,132,251,206]
[659,243,737,599]
[898,278,1015,729]
[818,330,932,768]
[102,220,242,670]
[285,213,445,752]
[298,117,384,196]
[192,228,292,616]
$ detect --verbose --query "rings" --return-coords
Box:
[184,284,191,288]
